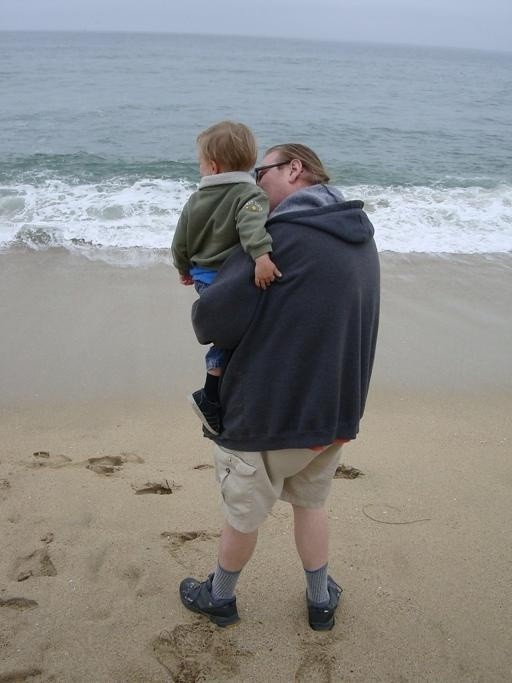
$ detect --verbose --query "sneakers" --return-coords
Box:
[179,573,241,627]
[187,389,221,437]
[306,575,339,631]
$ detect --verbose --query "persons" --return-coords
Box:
[179,143,381,632]
[171,122,282,436]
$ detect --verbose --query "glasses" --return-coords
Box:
[255,161,292,183]
